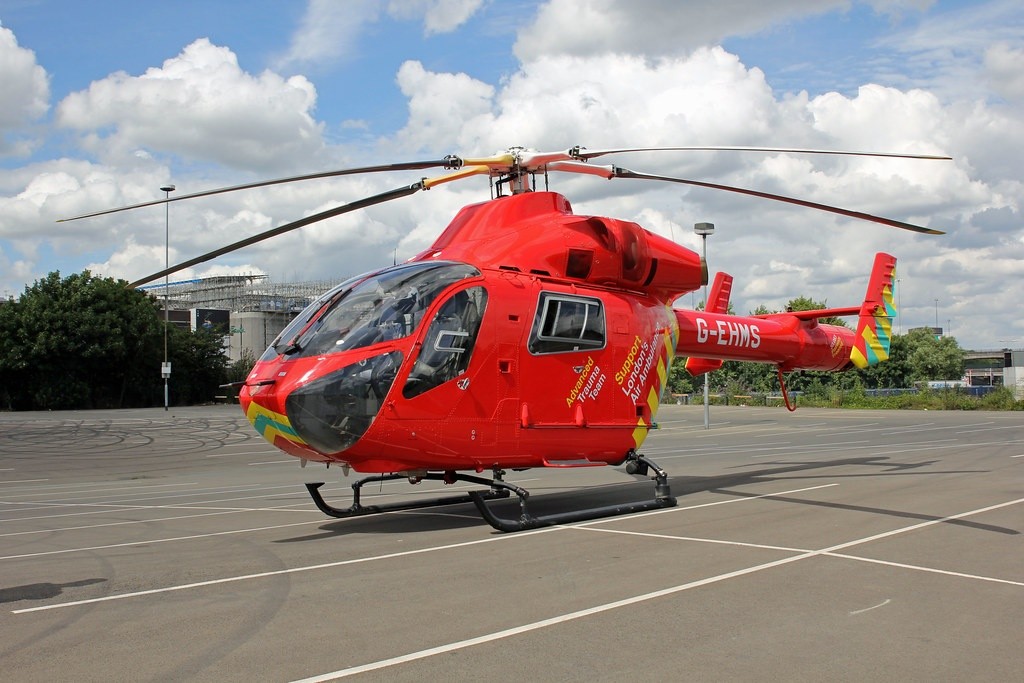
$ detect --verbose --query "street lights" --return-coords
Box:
[935,298,941,327]
[159,183,177,411]
[894,278,904,339]
[947,319,952,337]
[693,222,716,430]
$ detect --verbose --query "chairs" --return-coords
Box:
[407,288,463,378]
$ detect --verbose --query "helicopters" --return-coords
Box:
[56,142,956,534]
